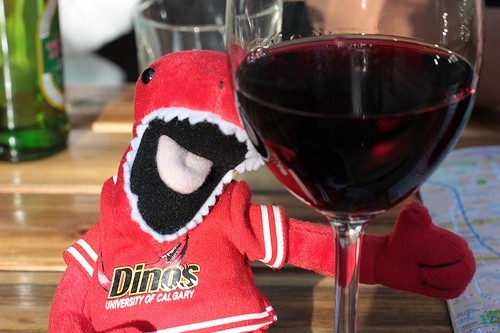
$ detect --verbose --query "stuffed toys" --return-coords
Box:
[48,44,476,333]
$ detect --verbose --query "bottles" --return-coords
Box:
[0,0,68,162]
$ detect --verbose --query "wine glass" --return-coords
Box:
[225,0,482,333]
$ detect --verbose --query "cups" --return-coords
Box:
[132,0,226,75]
